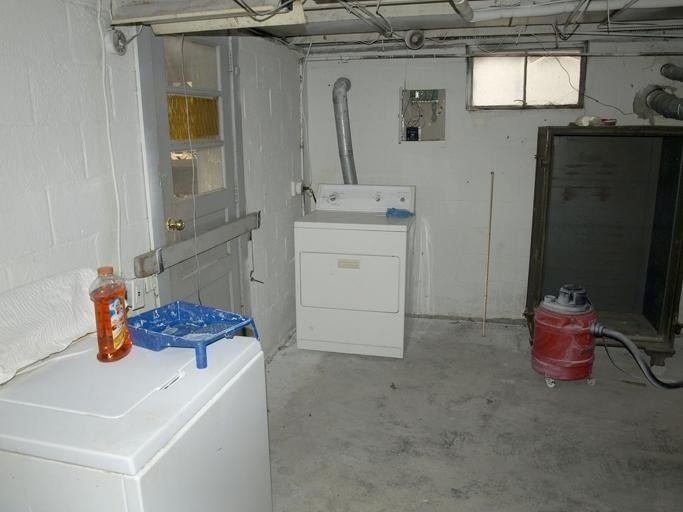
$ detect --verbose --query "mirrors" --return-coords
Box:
[402,90,446,142]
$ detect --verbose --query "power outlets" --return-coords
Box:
[126,277,146,310]
[291,180,304,197]
[106,30,126,57]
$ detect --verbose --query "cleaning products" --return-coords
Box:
[89,266,132,364]
